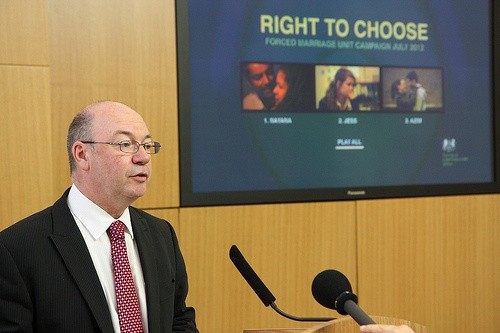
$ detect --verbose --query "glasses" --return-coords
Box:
[82,141,161,154]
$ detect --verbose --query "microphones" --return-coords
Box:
[311,269,377,326]
[229,245,337,322]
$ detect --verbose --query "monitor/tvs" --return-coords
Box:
[175,0,500,208]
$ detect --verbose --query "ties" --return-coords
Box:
[106,219,144,333]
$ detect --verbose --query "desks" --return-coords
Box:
[356,77,380,97]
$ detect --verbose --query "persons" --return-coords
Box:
[271,63,314,111]
[391,71,427,112]
[318,68,358,113]
[0,99,198,333]
[242,61,277,110]
